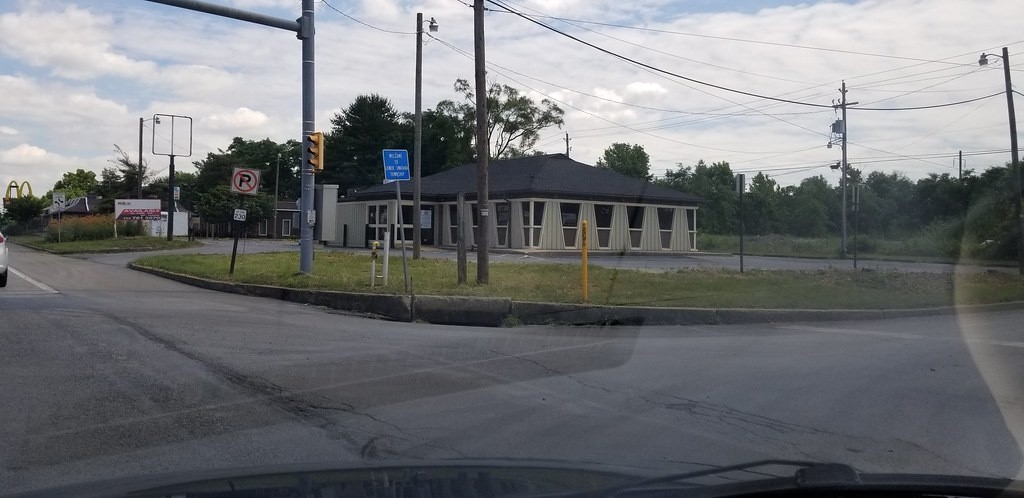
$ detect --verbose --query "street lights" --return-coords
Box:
[979,46,1024,274]
[138,116,160,199]
[274,153,282,239]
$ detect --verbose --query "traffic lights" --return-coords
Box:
[307,131,324,171]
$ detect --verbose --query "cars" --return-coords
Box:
[0,232,9,287]
